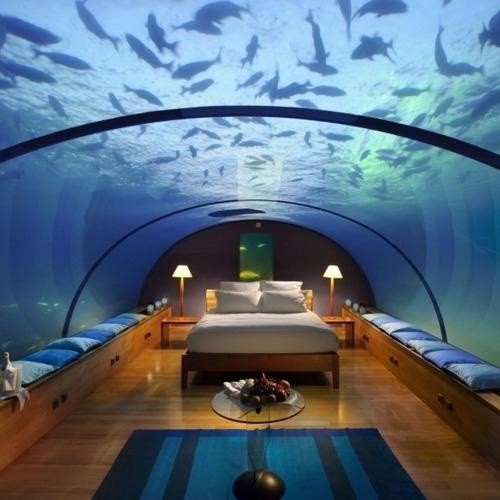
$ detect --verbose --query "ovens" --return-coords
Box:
[181,289,338,391]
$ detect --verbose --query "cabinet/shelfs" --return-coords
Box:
[2,352,13,370]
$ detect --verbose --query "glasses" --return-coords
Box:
[172,265,193,319]
[323,264,344,319]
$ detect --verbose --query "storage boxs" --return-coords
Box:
[0,303,173,471]
[340,308,500,472]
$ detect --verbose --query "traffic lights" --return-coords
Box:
[263,280,303,291]
[361,313,500,391]
[4,313,145,385]
[215,290,262,313]
[262,290,308,313]
[220,281,261,292]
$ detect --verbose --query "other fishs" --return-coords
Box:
[0,0,500,207]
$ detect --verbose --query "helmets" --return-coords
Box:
[265,389,298,406]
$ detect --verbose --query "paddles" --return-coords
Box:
[91,428,426,500]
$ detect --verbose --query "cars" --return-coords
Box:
[359,307,368,315]
[345,300,351,309]
[353,304,359,312]
[162,297,167,306]
[154,302,162,312]
[147,305,155,316]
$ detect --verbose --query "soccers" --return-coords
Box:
[241,369,292,405]
